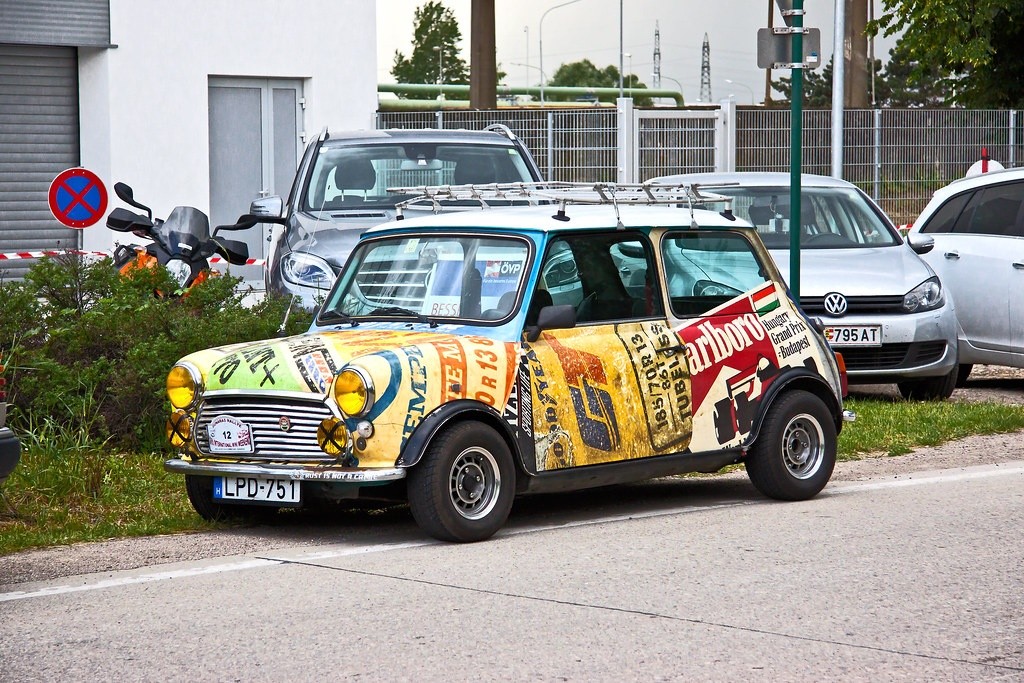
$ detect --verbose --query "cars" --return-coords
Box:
[903,168,1024,385]
[641,171,960,403]
[161,181,851,545]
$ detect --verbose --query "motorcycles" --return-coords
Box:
[105,181,257,310]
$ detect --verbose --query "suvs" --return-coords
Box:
[250,123,558,325]
